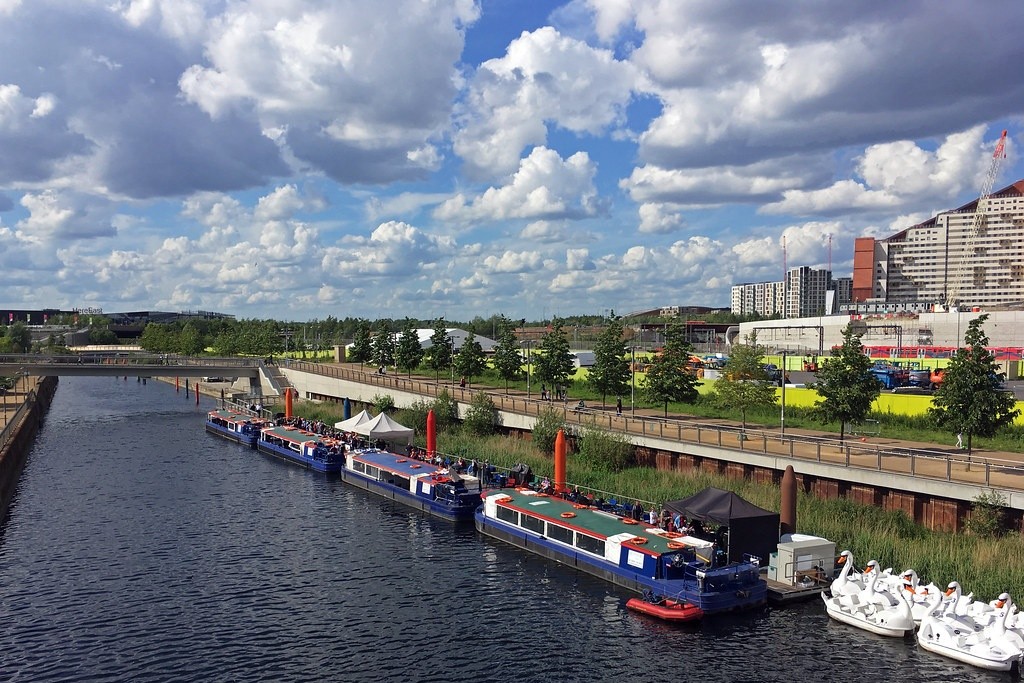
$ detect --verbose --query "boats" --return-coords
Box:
[473,486,768,617]
[626,586,703,621]
[256,425,350,475]
[339,447,494,523]
[819,549,1024,673]
[204,408,274,448]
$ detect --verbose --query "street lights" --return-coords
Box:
[446,336,460,389]
[389,332,402,377]
[772,350,796,445]
[624,346,643,423]
[520,339,537,405]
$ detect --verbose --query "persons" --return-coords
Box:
[616,399,622,415]
[78,353,83,364]
[287,415,490,487]
[265,357,267,365]
[540,382,562,402]
[160,355,163,364]
[268,354,275,365]
[382,364,386,375]
[538,476,551,494]
[579,399,584,407]
[250,404,255,411]
[500,473,506,487]
[460,376,465,390]
[569,485,728,550]
[255,403,262,418]
[165,353,169,365]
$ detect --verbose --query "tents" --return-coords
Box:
[334,409,375,438]
[664,487,780,566]
[353,411,414,450]
